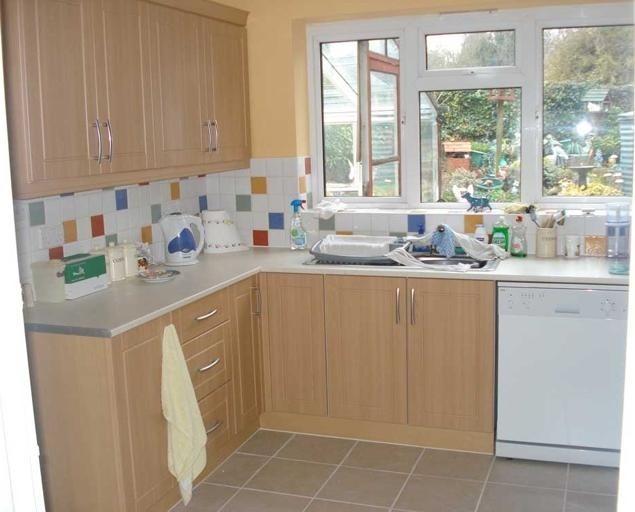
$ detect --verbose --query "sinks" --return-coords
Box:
[415,255,483,272]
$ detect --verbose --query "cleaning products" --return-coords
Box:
[510,214,527,257]
[491,216,509,257]
[289,197,307,249]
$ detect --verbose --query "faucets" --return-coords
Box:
[437,225,445,234]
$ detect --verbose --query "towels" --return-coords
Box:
[161,323,209,506]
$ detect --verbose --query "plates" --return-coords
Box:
[139,270,181,283]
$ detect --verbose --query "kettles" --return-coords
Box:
[160,212,205,266]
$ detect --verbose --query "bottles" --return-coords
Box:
[605,202,629,275]
[536,227,557,258]
[474,225,489,244]
[107,242,125,282]
[119,240,138,279]
[565,235,580,258]
[89,245,111,285]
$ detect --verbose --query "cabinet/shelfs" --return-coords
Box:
[148,0,252,183]
[324,274,496,455]
[227,272,259,448]
[259,271,327,436]
[24,282,235,512]
[0,0,154,200]
